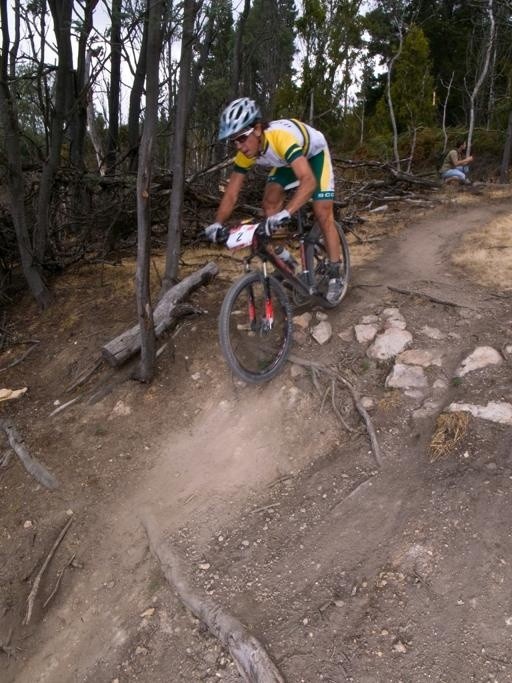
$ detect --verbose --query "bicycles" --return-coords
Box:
[190,181,352,385]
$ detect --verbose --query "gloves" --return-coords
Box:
[204,222,224,245]
[264,209,291,237]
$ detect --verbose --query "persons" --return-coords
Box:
[203,97,349,303]
[438,139,474,184]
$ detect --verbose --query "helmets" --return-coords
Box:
[217,97,262,141]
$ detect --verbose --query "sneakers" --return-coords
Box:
[271,253,299,281]
[326,276,347,306]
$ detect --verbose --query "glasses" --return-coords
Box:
[228,126,254,146]
